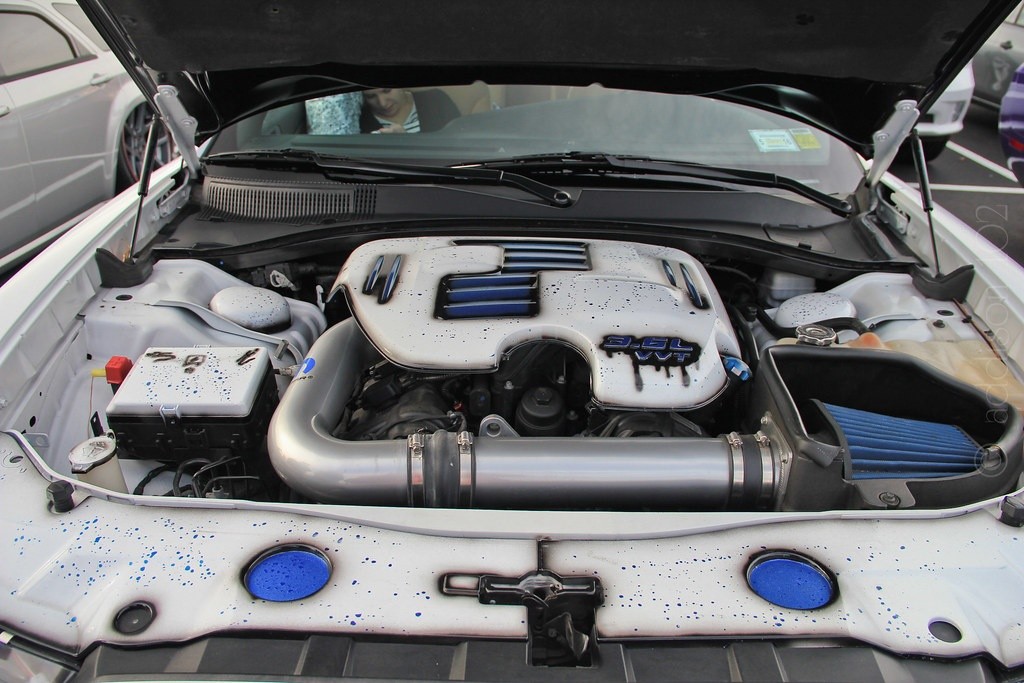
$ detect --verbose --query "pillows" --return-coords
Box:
[304,91,360,135]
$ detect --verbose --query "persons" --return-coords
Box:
[361,87,461,134]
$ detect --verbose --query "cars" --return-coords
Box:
[0,1,183,270]
[0,0,1024,683]
[889,0,1024,184]
